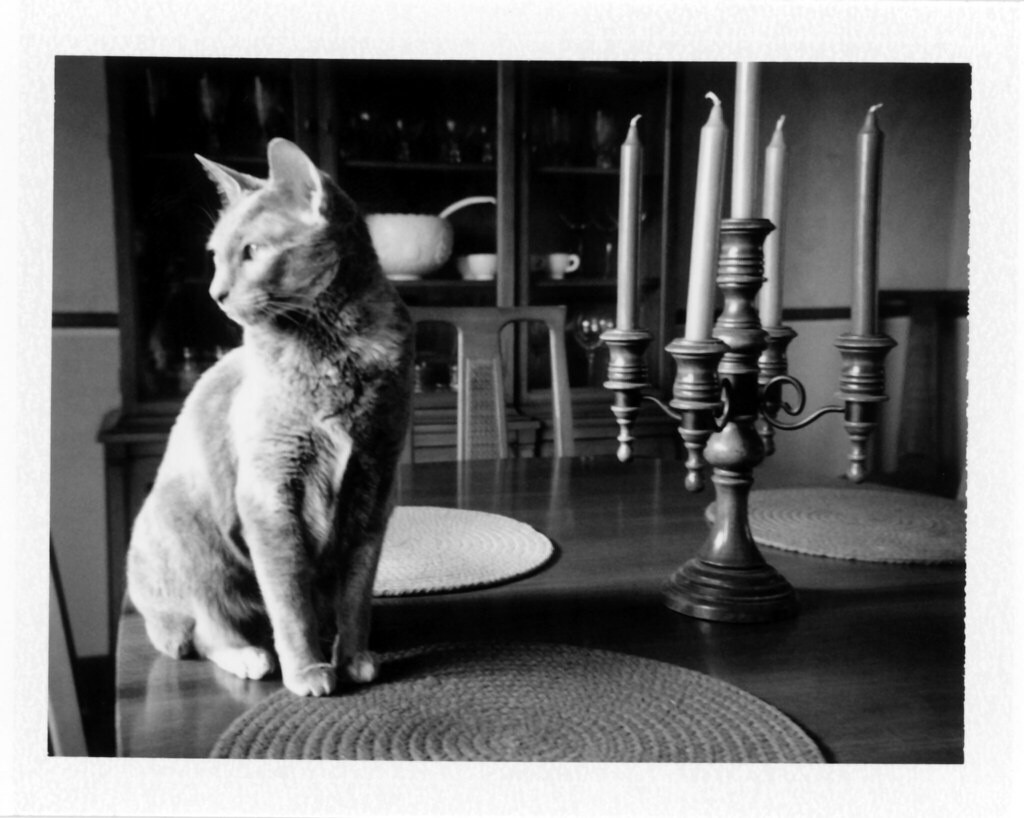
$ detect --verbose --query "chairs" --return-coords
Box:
[398,304,575,464]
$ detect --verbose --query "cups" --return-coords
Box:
[528,252,580,280]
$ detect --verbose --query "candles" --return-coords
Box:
[614,114,643,330]
[758,115,790,328]
[682,92,727,340]
[851,103,886,335]
[730,63,763,219]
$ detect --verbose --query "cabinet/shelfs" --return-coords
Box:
[99,56,686,658]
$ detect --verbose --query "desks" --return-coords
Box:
[114,453,965,766]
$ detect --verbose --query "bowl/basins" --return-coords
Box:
[458,254,497,281]
[363,214,454,282]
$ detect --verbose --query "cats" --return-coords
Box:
[126,136,417,697]
